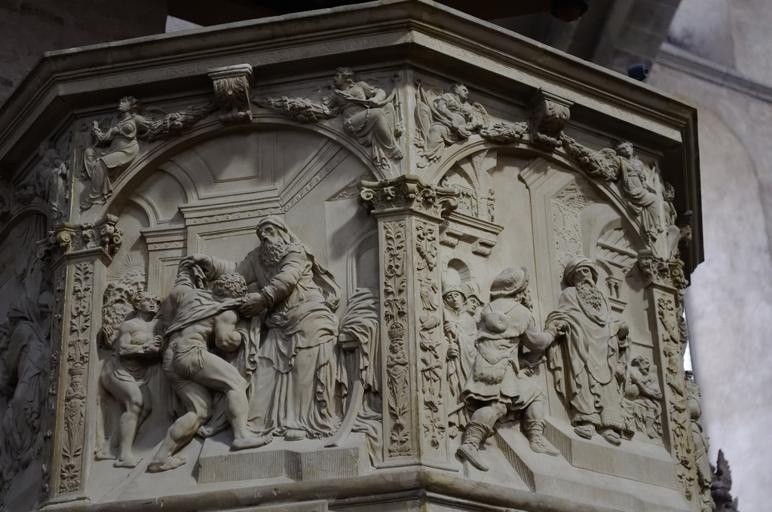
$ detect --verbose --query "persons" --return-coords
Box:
[464,280,484,319]
[413,80,484,171]
[548,256,636,448]
[97,280,141,348]
[187,214,343,443]
[456,264,564,472]
[442,279,479,430]
[617,138,681,266]
[314,67,406,169]
[92,288,165,471]
[619,353,665,441]
[82,94,163,216]
[148,253,275,473]
